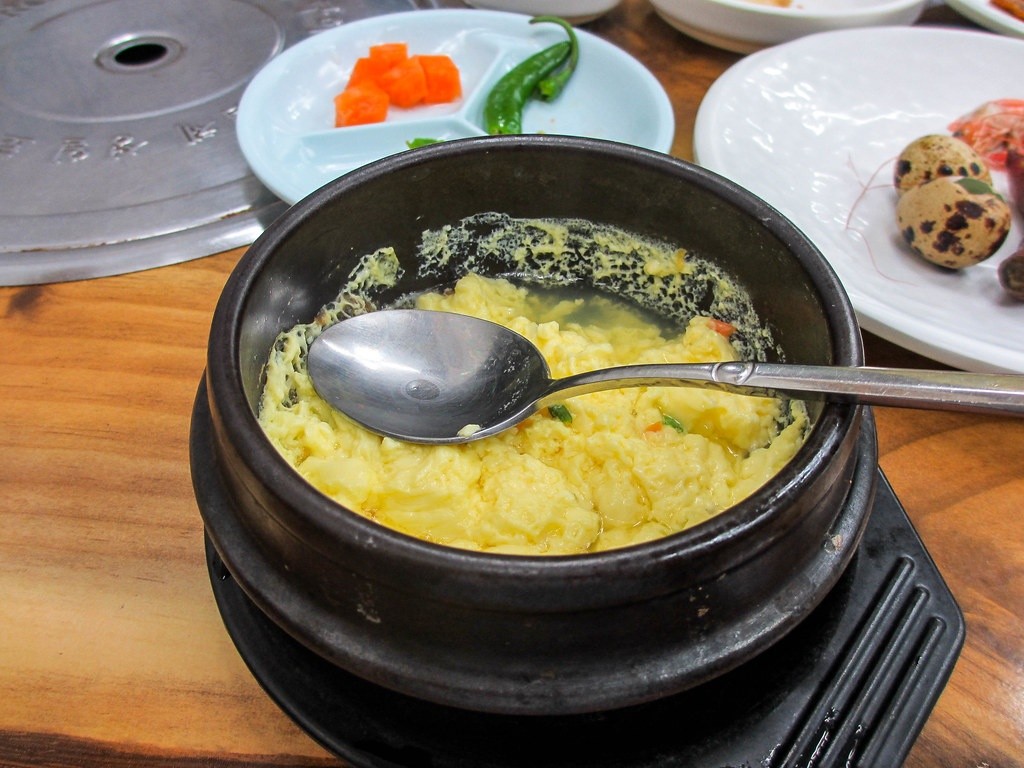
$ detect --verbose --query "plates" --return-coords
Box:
[691,26,1024,379]
[234,8,675,206]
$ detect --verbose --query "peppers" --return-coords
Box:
[484,43,571,137]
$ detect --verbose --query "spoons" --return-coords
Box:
[305,309,1024,446]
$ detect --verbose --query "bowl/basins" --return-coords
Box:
[189,133,879,721]
